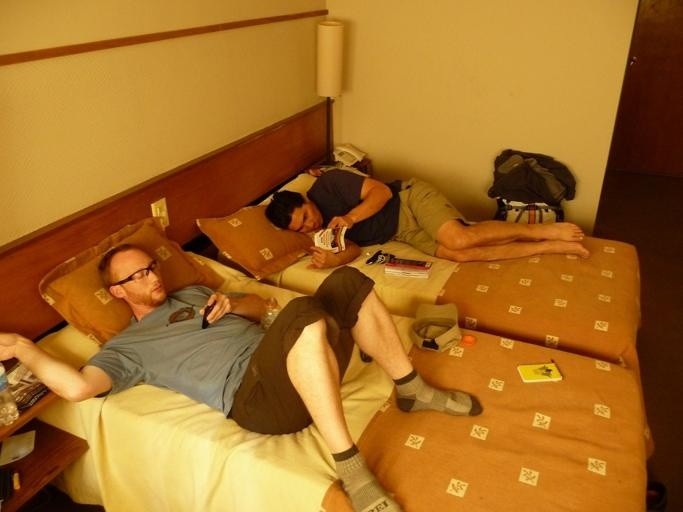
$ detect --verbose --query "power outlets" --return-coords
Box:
[151,197,170,228]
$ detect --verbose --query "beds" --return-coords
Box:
[218,172,642,373]
[7,251,656,512]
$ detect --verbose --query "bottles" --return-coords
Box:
[260,296,282,328]
[0,363,20,426]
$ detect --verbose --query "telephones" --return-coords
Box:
[333,144,366,167]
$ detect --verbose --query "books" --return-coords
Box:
[517,363,564,383]
[385,258,432,279]
[312,226,347,254]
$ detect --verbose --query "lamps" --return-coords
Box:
[317,21,345,165]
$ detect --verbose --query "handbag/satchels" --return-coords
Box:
[488,149,576,201]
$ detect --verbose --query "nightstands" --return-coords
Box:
[304,154,371,178]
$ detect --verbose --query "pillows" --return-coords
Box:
[38,218,225,349]
[197,205,315,281]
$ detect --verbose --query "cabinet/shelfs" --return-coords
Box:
[0,391,88,512]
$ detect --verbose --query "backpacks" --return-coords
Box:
[493,198,564,223]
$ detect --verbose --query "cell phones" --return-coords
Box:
[202,300,217,328]
[366,250,382,264]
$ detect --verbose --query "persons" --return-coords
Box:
[265,170,590,262]
[1,244,483,512]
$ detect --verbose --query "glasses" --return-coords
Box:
[109,261,157,286]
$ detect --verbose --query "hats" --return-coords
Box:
[411,303,463,353]
